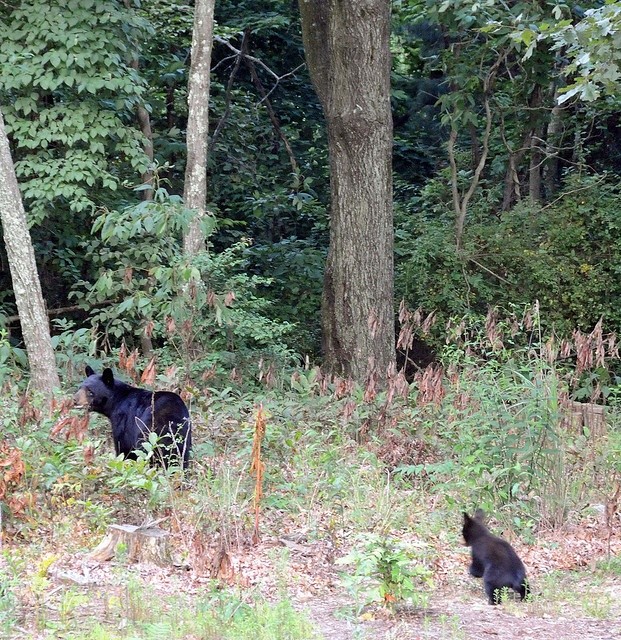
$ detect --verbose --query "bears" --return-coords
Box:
[74,366,191,479]
[464,509,529,605]
